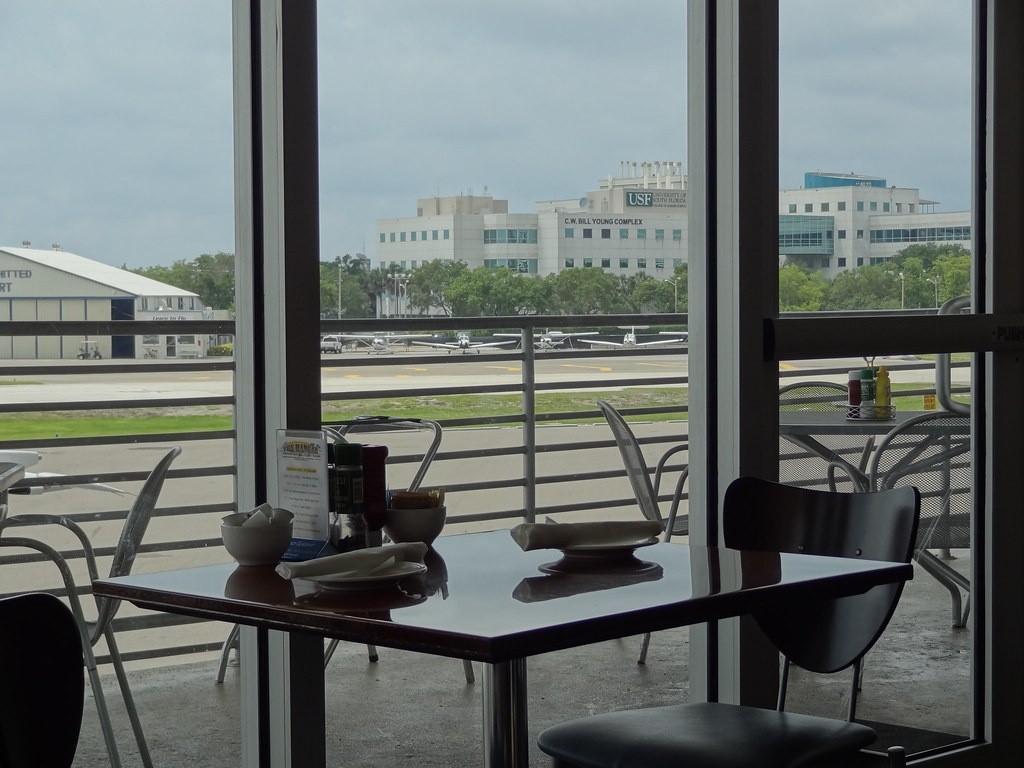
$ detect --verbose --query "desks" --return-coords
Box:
[778,412,970,629]
[93,529,915,768]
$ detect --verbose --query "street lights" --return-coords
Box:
[399,279,410,319]
[899,272,905,308]
[926,275,942,308]
[664,276,681,314]
[386,273,412,319]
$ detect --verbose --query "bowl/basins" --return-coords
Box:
[222,523,292,566]
[384,508,446,546]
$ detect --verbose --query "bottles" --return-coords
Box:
[860,370,876,419]
[328,444,336,512]
[848,371,861,418]
[334,444,365,514]
[875,366,890,418]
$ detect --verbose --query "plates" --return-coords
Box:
[301,560,427,591]
[294,590,427,613]
[538,558,658,577]
[560,536,660,557]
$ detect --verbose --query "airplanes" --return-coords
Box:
[577,325,684,349]
[329,334,439,355]
[493,330,599,350]
[658,331,689,344]
[411,331,517,354]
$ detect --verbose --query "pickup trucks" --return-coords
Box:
[321,336,343,354]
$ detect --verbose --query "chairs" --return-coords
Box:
[0,377,970,767]
